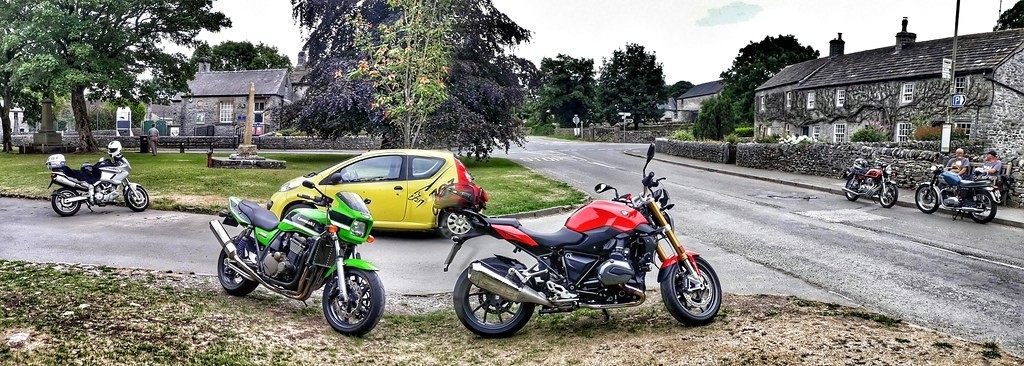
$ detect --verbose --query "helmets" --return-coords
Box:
[854,158,867,169]
[107,140,122,157]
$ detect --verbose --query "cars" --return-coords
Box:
[265,149,482,239]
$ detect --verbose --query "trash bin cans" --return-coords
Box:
[140,135,149,153]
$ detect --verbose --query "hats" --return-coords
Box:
[987,151,997,158]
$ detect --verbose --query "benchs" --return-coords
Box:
[937,163,1011,207]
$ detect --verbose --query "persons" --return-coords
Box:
[981,151,1002,183]
[946,148,973,181]
[148,124,160,155]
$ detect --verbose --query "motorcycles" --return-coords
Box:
[46,145,149,217]
[915,163,1002,224]
[432,142,722,340]
[842,160,899,208]
[208,173,386,338]
